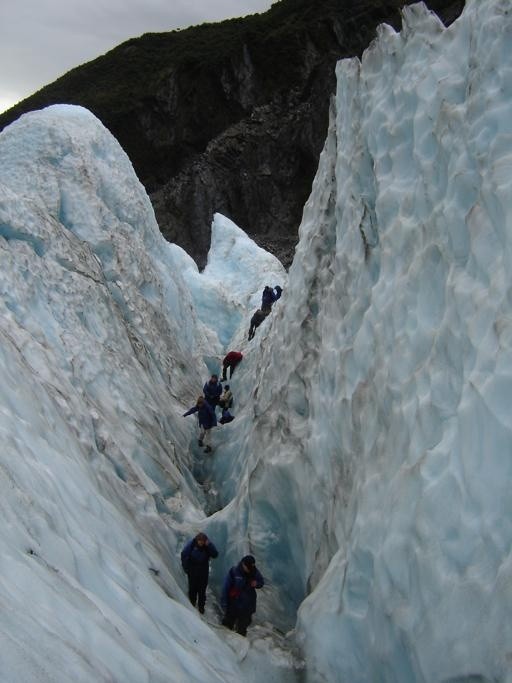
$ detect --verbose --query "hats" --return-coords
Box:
[243,556,257,576]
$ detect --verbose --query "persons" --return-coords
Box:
[219,384,233,420]
[221,555,264,637]
[181,396,217,453]
[220,350,242,382]
[181,532,219,614]
[248,309,265,341]
[203,374,223,412]
[260,285,283,315]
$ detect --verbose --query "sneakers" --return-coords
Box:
[204,446,211,452]
[198,439,203,447]
